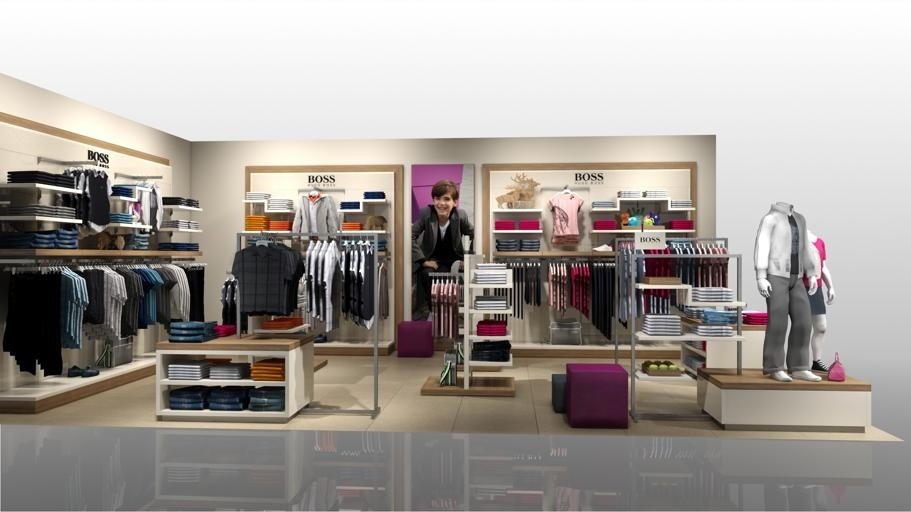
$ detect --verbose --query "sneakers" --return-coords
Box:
[67,365,100,378]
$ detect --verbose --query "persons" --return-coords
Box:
[754,201,823,383]
[410,180,475,320]
[806,229,835,371]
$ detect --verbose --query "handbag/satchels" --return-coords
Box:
[827,350,846,382]
